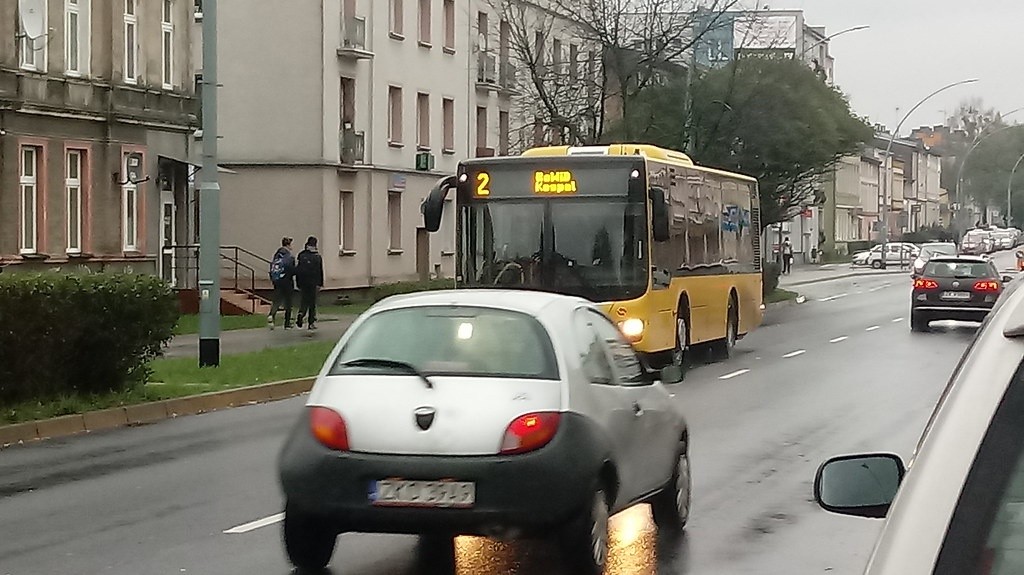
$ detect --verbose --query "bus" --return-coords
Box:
[421,144,764,373]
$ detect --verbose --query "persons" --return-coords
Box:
[267,236,296,331]
[780,237,793,274]
[294,237,324,329]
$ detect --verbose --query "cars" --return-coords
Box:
[852,250,869,266]
[958,233,987,259]
[988,223,1024,253]
[980,231,996,254]
[915,243,961,275]
[277,288,694,573]
[909,256,1014,334]
[866,244,921,270]
[814,270,1024,575]
[900,242,921,258]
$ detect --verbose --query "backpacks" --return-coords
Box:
[270,253,287,283]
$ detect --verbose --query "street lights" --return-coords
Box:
[955,105,1024,233]
[882,77,979,269]
[778,23,871,271]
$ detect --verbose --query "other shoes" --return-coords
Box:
[267,315,274,331]
[296,312,303,328]
[307,324,317,332]
[285,321,292,330]
